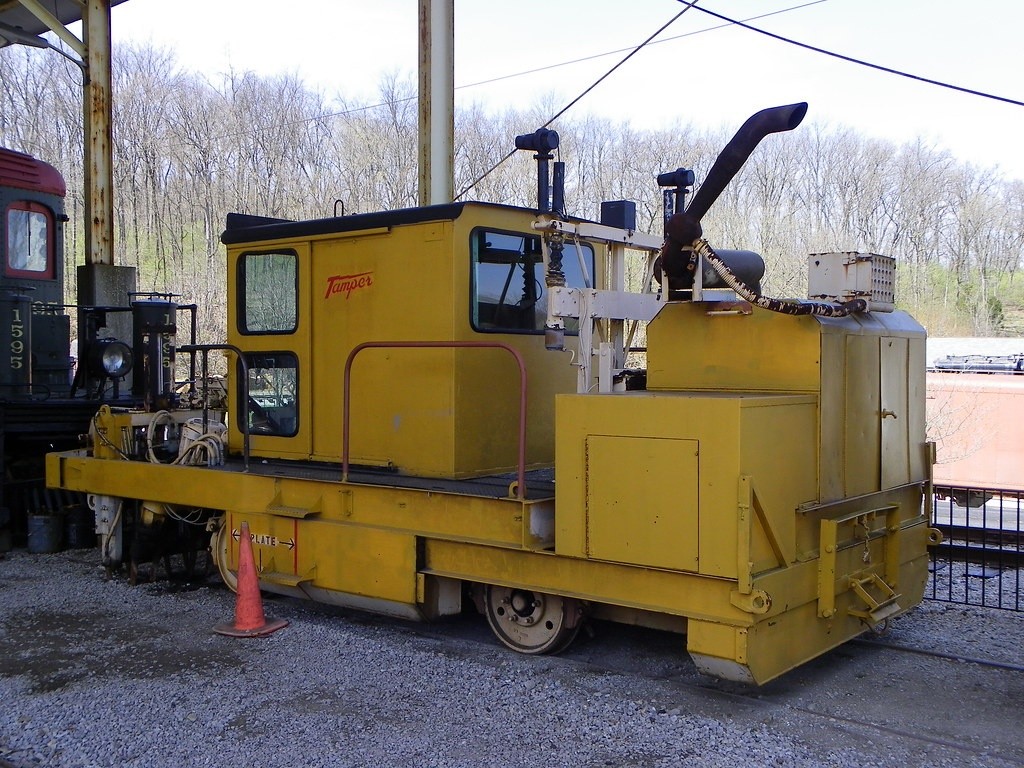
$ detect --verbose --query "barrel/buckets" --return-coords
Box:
[27,510,62,554]
[62,503,96,550]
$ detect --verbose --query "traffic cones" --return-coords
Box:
[209,519,291,640]
